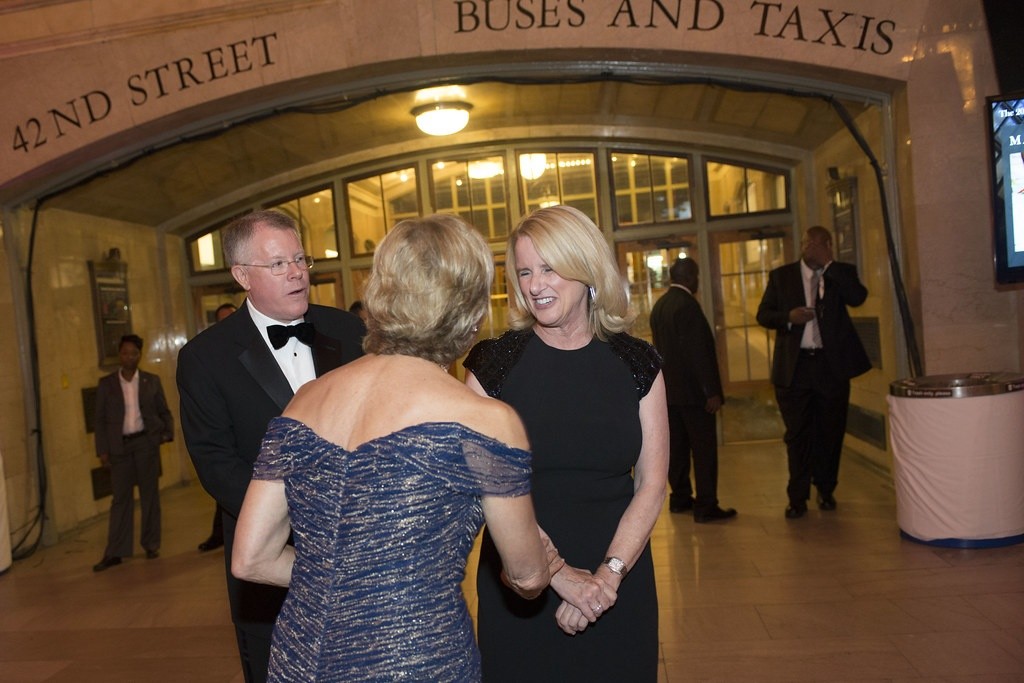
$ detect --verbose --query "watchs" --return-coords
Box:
[603,557,628,577]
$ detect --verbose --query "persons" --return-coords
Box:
[176,210,368,683]
[461,205,671,683]
[93,334,174,572]
[197,303,239,552]
[756,226,874,519]
[231,213,566,683]
[650,257,738,523]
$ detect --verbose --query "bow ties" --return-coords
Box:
[266,318,316,350]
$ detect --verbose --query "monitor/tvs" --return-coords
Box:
[986,92,1024,285]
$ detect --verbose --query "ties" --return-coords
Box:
[811,270,825,349]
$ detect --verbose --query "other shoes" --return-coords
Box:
[669,493,695,512]
[785,498,807,518]
[93,558,122,571]
[815,488,836,511]
[199,535,224,551]
[694,508,737,524]
[147,549,159,559]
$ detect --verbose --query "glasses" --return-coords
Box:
[233,254,316,276]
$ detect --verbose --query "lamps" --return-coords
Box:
[409,101,474,138]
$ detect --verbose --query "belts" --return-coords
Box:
[124,430,148,441]
[798,348,825,357]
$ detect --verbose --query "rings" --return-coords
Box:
[593,604,602,612]
